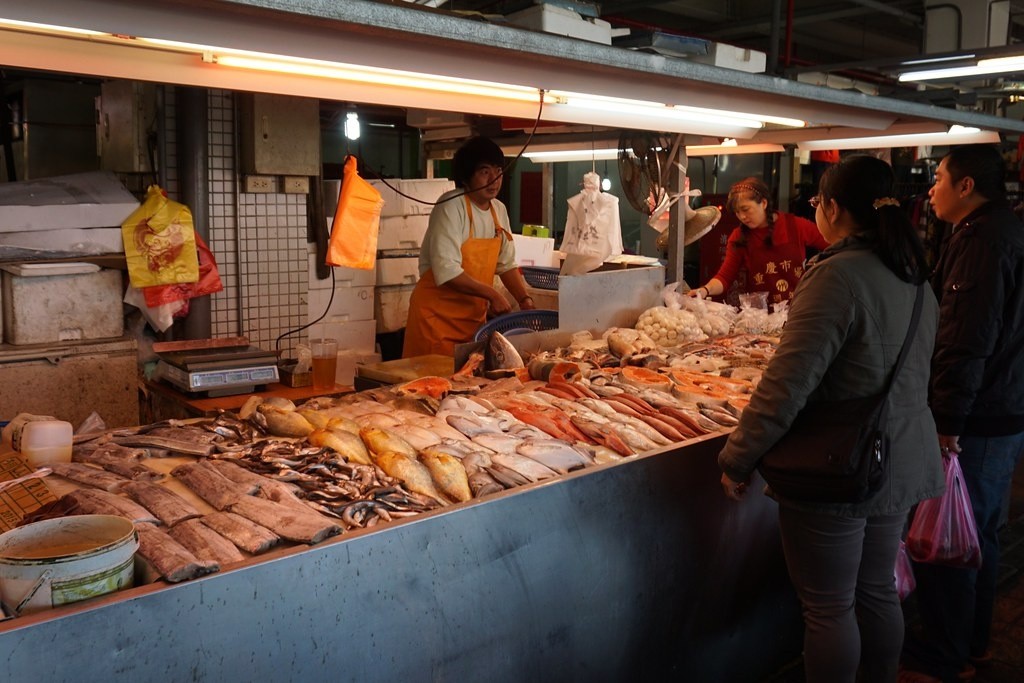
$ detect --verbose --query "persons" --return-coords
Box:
[718,155,946,683]
[901,143,1024,683]
[402,139,538,358]
[686,175,829,314]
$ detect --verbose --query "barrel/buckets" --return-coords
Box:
[0,514,139,617]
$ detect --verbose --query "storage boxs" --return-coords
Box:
[509,3,612,45]
[308,178,554,385]
[692,43,766,74]
[0,263,123,345]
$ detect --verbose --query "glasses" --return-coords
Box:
[808,194,840,209]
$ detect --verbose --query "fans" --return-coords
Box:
[618,130,670,214]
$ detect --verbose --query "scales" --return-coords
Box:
[154,344,283,398]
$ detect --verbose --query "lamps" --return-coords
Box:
[0,0,1024,162]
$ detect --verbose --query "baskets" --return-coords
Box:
[473,309,559,342]
[519,267,559,291]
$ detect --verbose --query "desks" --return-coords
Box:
[145,379,356,422]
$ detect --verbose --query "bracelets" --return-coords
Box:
[703,286,710,296]
[520,295,534,306]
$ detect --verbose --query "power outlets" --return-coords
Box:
[283,177,309,193]
[246,175,277,193]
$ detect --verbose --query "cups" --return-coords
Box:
[311,339,339,389]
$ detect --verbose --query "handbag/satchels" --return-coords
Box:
[906,448,982,568]
[756,393,890,506]
[893,544,915,599]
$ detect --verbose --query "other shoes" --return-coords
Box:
[967,624,993,668]
[901,639,976,683]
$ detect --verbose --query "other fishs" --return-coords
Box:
[52,418,342,584]
[257,397,590,506]
[194,405,421,529]
[485,330,741,458]
